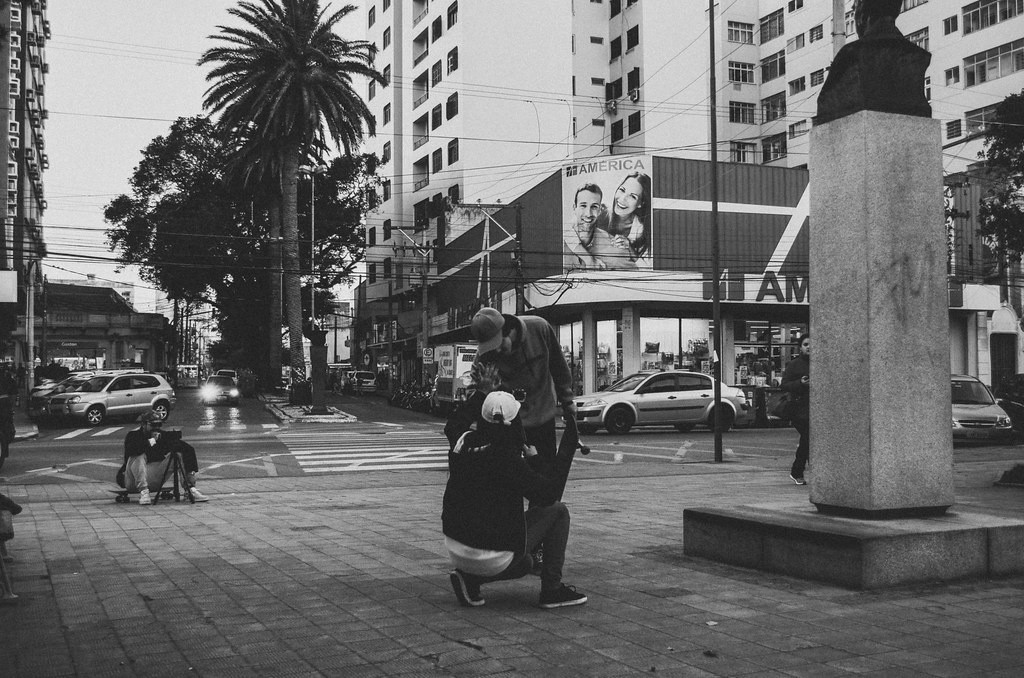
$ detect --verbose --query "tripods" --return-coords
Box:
[152,451,195,505]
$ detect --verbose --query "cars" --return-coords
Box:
[950,373,1012,440]
[341,371,377,394]
[559,369,749,435]
[47,374,177,426]
[26,369,145,417]
[202,376,239,406]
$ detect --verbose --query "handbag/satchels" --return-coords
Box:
[774,391,802,420]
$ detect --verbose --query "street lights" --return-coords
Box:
[299,164,328,330]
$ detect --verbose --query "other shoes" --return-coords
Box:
[532,548,543,575]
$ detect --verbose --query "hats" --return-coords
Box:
[142,410,163,423]
[471,307,505,357]
[482,391,522,427]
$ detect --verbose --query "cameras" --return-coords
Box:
[150,429,183,450]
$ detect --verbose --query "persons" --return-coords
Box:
[563,171,653,272]
[343,371,364,397]
[116,410,209,505]
[783,332,811,482]
[440,306,594,611]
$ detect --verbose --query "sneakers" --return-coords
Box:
[539,584,587,609]
[450,569,485,606]
[790,472,806,486]
[185,487,209,502]
[139,488,152,505]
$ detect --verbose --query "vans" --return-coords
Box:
[217,370,237,386]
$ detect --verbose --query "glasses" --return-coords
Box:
[148,423,163,426]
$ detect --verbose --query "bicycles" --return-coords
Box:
[391,378,433,413]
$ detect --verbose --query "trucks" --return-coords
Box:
[428,343,478,417]
